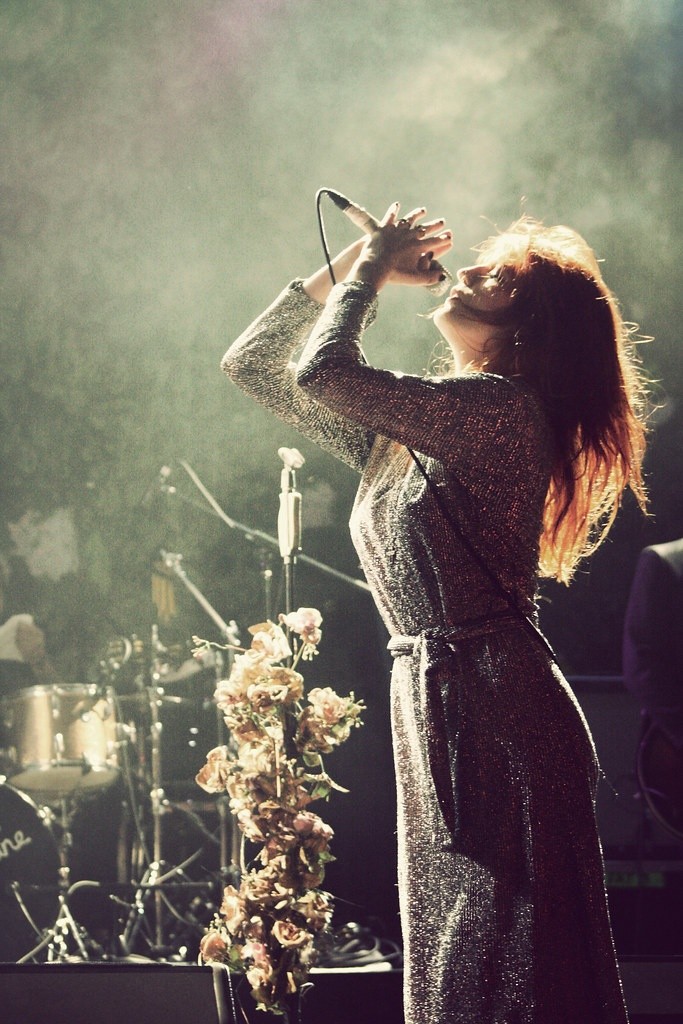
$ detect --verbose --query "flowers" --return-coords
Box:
[201,604,366,1018]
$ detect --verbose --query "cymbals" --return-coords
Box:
[115,691,194,709]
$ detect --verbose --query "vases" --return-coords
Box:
[213,964,290,1024]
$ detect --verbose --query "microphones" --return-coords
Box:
[327,189,453,296]
[137,463,174,510]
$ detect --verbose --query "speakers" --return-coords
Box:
[567,672,683,860]
[298,967,407,1024]
[0,962,237,1024]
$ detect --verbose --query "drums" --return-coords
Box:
[0,682,123,794]
[0,782,67,962]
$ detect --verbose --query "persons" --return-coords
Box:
[219,201,635,1024]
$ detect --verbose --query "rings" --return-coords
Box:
[399,217,410,228]
[414,224,427,237]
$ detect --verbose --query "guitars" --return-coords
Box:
[636,718,683,845]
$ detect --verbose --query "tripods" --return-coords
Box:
[22,619,219,964]
